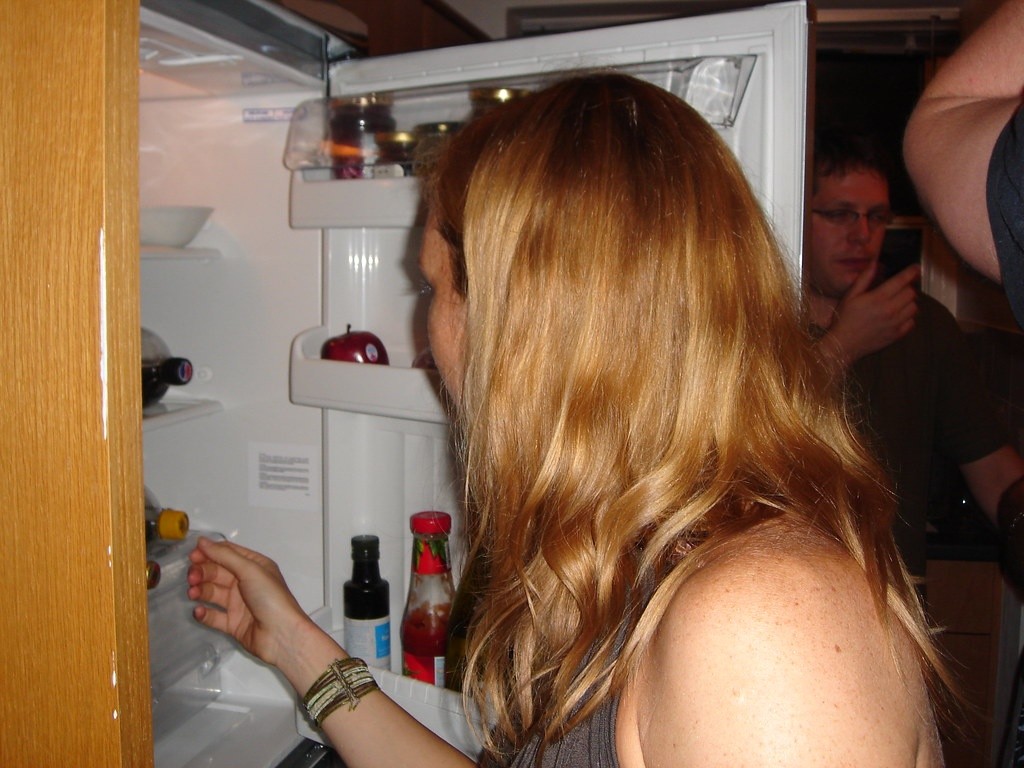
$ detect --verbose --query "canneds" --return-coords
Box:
[328,87,530,179]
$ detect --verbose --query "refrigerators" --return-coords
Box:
[137,0,805,767]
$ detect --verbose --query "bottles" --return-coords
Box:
[343,534,391,672]
[144,485,189,542]
[399,510,457,685]
[141,328,193,410]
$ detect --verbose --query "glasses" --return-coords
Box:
[813,209,894,225]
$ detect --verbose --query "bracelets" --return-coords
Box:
[301,654,378,728]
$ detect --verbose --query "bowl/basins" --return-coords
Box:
[139,205,214,247]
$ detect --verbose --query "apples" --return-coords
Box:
[320,323,389,368]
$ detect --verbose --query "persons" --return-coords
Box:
[807,1,1024,768]
[187,74,950,767]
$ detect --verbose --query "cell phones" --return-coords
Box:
[869,228,921,291]
